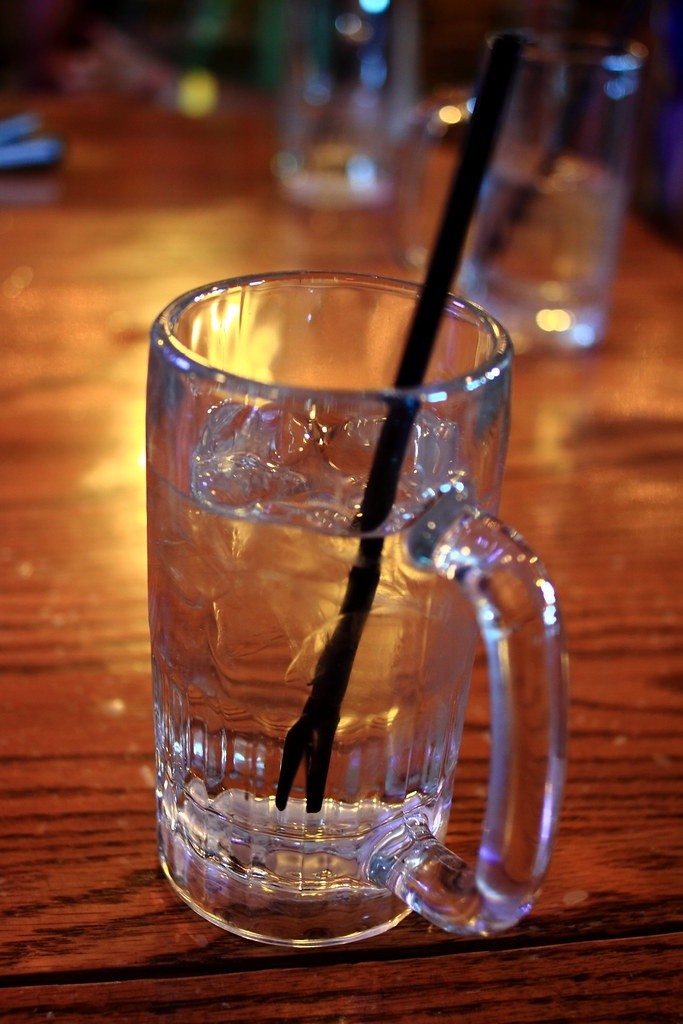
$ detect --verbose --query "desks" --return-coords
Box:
[0,86,682,1024]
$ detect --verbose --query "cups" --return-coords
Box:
[261,2,422,210]
[460,32,645,353]
[143,268,564,946]
